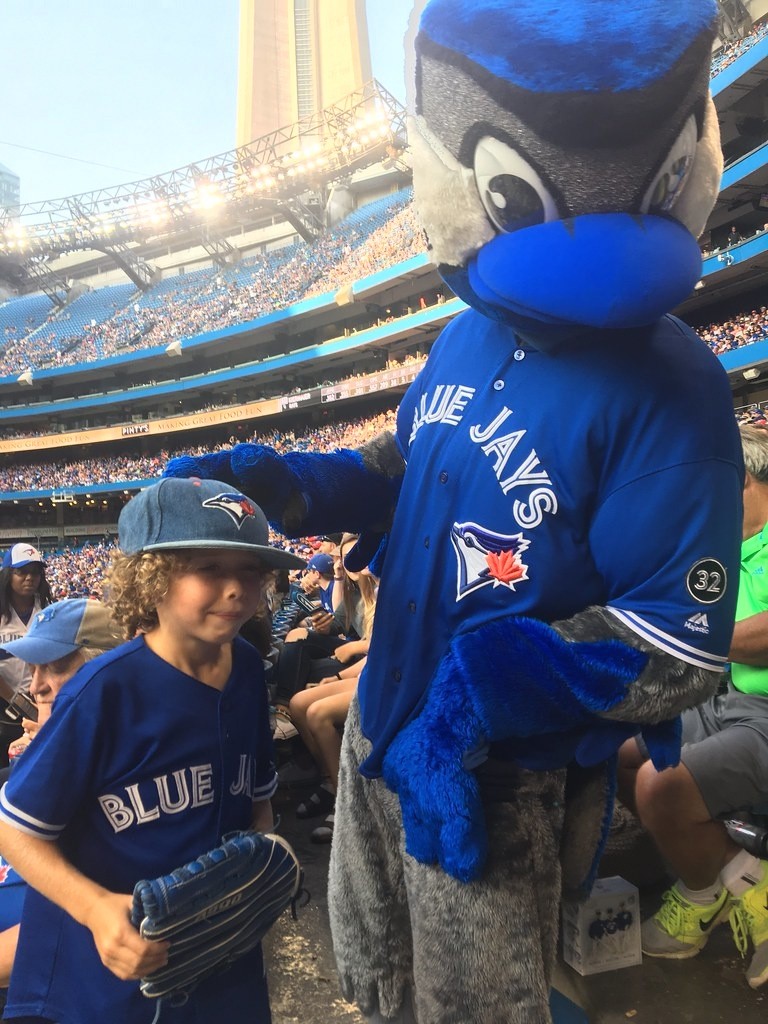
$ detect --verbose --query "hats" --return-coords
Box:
[2,542,47,569]
[117,476,308,570]
[0,598,126,665]
[0,551,3,558]
[306,553,334,572]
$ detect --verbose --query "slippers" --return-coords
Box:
[309,808,336,844]
[296,786,335,820]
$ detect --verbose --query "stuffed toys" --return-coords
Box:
[162,0,745,1024]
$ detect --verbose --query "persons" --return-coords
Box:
[0,24,768,1024]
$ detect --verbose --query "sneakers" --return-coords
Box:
[276,763,303,781]
[728,858,768,989]
[636,885,733,960]
[272,718,299,742]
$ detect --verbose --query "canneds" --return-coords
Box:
[722,818,768,852]
